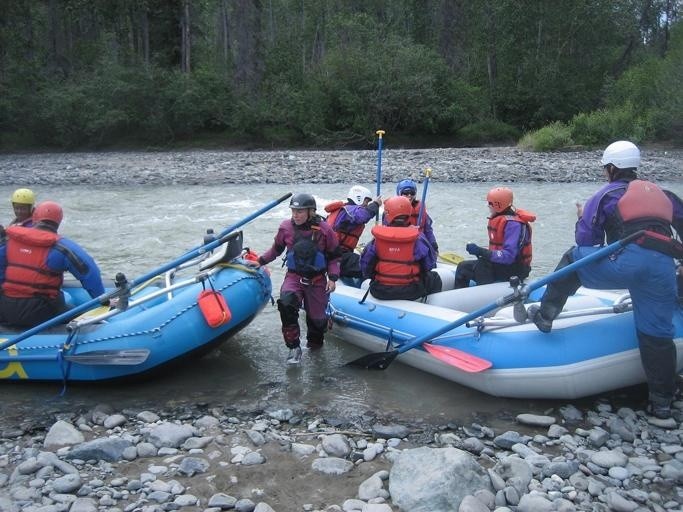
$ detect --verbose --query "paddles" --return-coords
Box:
[68,275,160,327]
[343,230,646,370]
[436,252,464,264]
[327,311,492,374]
[0,349,150,364]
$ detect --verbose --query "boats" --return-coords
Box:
[0,247,272,382]
[326,256,682,399]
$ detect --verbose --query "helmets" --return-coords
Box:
[396,178,418,198]
[32,201,64,226]
[383,196,413,225]
[10,188,35,213]
[598,139,643,173]
[486,185,514,214]
[346,185,374,207]
[288,191,317,211]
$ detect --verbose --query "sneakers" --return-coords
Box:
[642,399,672,420]
[525,303,553,334]
[285,345,303,366]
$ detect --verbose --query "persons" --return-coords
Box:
[454,188,532,290]
[325,186,383,278]
[0,201,119,328]
[360,196,442,300]
[525,139,683,419]
[246,193,342,365]
[382,180,439,267]
[0,188,36,237]
